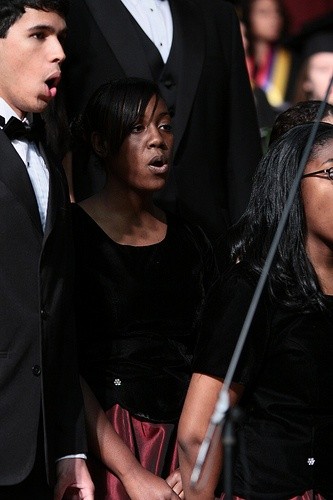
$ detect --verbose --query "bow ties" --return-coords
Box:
[3,117,48,141]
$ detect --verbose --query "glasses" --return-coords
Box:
[302,167,333,179]
